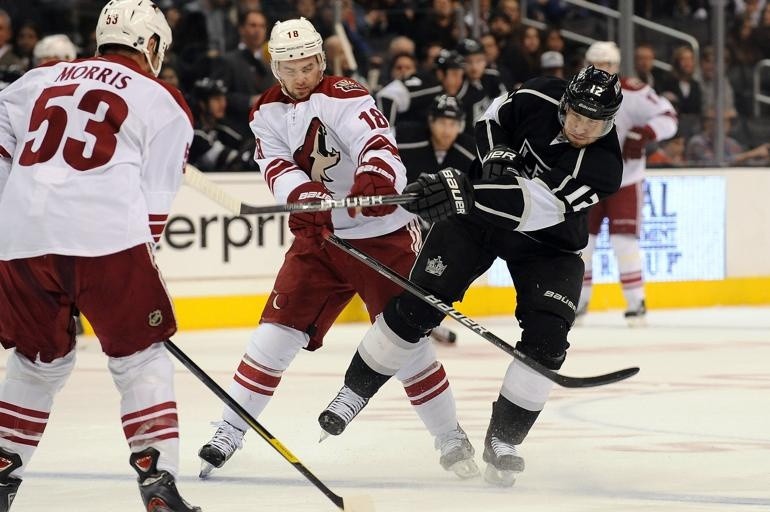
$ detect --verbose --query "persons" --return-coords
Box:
[0,0,200,511]
[395,95,476,342]
[317,64,624,470]
[571,40,679,316]
[198,15,475,471]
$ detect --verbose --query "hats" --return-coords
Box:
[540,50,566,70]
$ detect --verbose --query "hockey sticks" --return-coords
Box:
[319,227,639,387]
[166,339,422,512]
[184,164,421,217]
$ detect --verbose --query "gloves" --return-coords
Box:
[284,180,338,255]
[400,165,478,226]
[476,142,528,188]
[344,159,402,218]
[621,123,650,164]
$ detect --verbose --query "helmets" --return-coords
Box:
[264,15,332,86]
[94,0,174,78]
[430,47,468,70]
[583,39,623,67]
[428,93,466,122]
[190,76,231,101]
[562,61,628,124]
[455,38,488,58]
[32,32,79,65]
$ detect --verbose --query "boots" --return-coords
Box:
[128,446,205,512]
[481,400,532,475]
[0,445,31,512]
[316,383,374,440]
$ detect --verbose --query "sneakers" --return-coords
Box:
[622,297,649,317]
[432,420,477,474]
[193,417,249,470]
